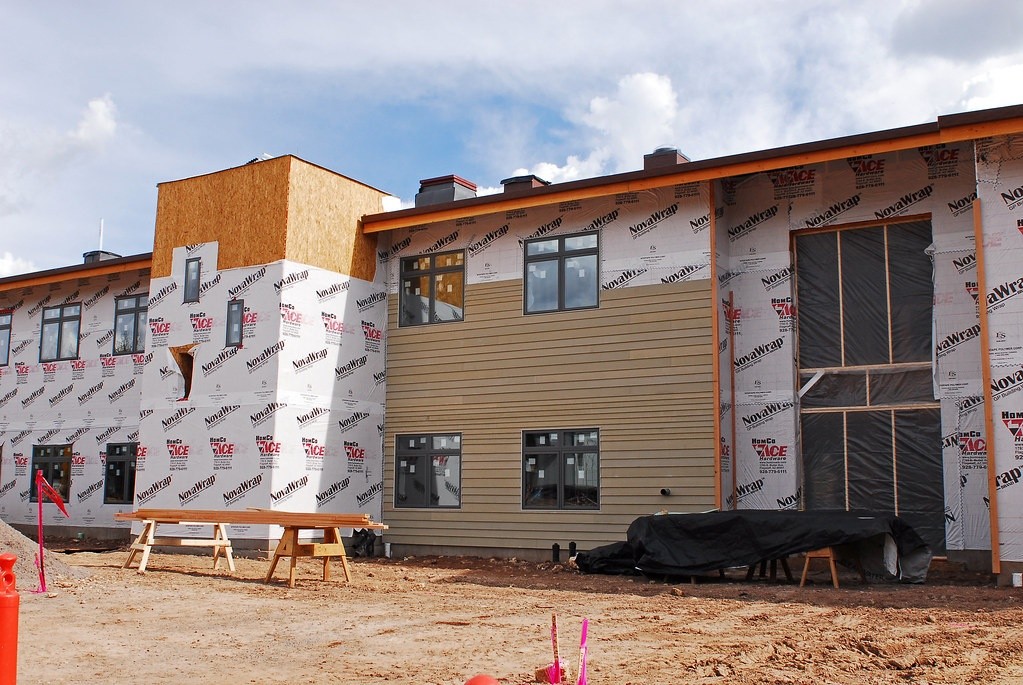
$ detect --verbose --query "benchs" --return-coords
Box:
[801,548,868,589]
[122,520,237,572]
[263,526,351,589]
